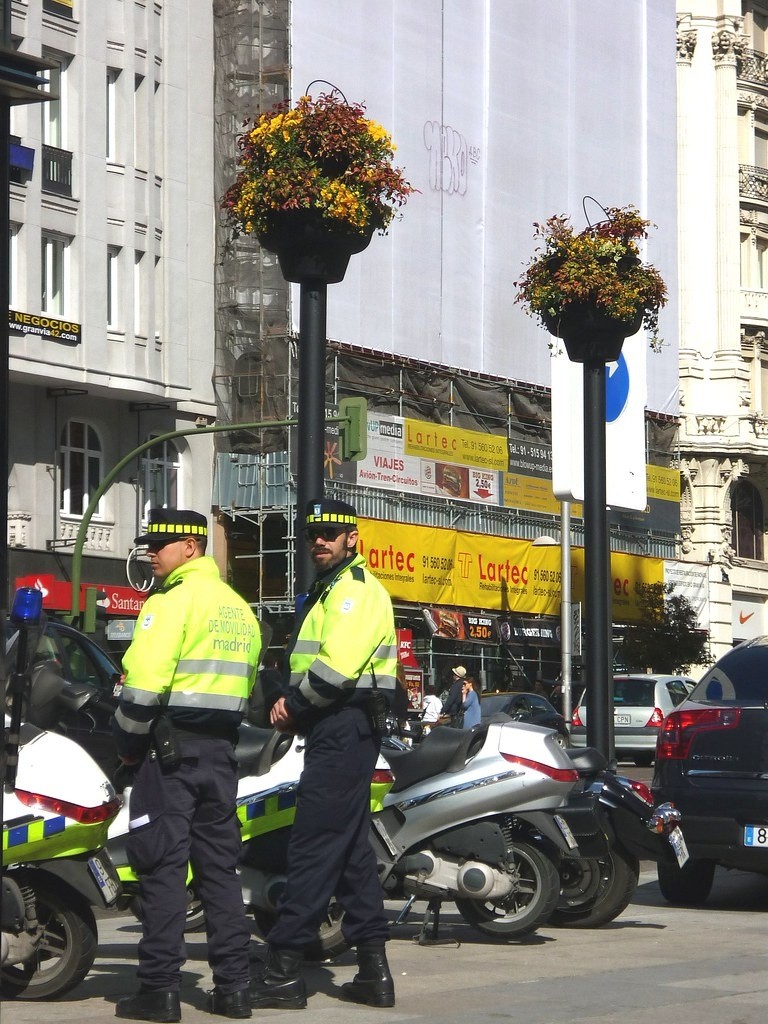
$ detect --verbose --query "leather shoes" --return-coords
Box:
[205,987,252,1018]
[116,989,181,1022]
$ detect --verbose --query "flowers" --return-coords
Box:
[513,203,670,356]
[213,87,423,265]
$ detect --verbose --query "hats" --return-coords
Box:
[452,666,466,678]
[300,497,357,528]
[133,508,207,544]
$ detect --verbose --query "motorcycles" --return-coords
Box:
[0,586,681,1002]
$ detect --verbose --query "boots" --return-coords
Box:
[248,945,306,1010]
[341,943,395,1007]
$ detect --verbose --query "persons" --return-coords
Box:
[440,665,467,728]
[104,508,266,1024]
[531,680,549,700]
[462,676,482,731]
[269,499,400,1009]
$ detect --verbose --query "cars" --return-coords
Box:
[481,692,571,749]
[568,673,699,767]
[649,634,768,905]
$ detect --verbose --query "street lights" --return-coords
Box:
[532,536,571,734]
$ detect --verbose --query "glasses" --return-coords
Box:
[304,528,349,542]
[463,682,469,685]
[149,537,200,552]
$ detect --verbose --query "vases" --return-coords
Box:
[541,256,644,364]
[255,156,374,284]
